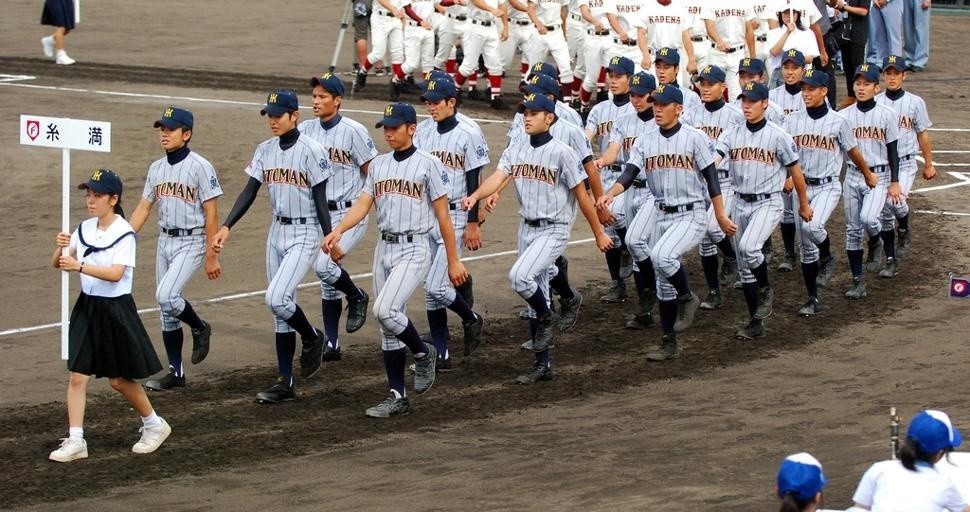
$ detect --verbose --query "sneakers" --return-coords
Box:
[365,392,411,419]
[752,285,775,318]
[600,279,628,303]
[50,440,89,462]
[409,340,452,395]
[626,312,656,328]
[351,55,613,120]
[672,290,700,331]
[132,418,171,454]
[640,286,657,313]
[619,244,634,278]
[799,295,821,316]
[300,327,342,379]
[719,258,734,286]
[557,288,582,332]
[646,342,677,359]
[735,274,744,289]
[256,381,296,403]
[189,320,213,366]
[144,374,188,392]
[735,323,768,341]
[815,255,837,288]
[345,288,370,333]
[460,312,484,358]
[849,229,910,300]
[778,251,797,270]
[905,62,926,72]
[516,303,558,384]
[40,35,75,64]
[700,290,724,309]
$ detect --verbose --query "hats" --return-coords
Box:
[153,104,195,131]
[608,47,907,106]
[520,62,561,117]
[419,71,458,103]
[260,90,299,119]
[75,169,124,199]
[307,71,347,96]
[777,452,827,504]
[375,103,417,128]
[907,409,965,455]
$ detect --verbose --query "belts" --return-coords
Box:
[633,178,647,190]
[686,33,771,52]
[658,203,693,214]
[899,154,909,163]
[161,227,197,235]
[449,203,463,209]
[374,8,637,49]
[523,213,556,228]
[276,215,314,225]
[848,163,886,173]
[381,233,414,244]
[734,191,769,203]
[606,164,621,172]
[325,201,353,210]
[805,176,831,186]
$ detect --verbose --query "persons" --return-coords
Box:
[296,72,380,363]
[38,0,81,65]
[850,409,970,511]
[47,167,174,462]
[127,107,224,393]
[774,449,866,511]
[211,90,347,403]
